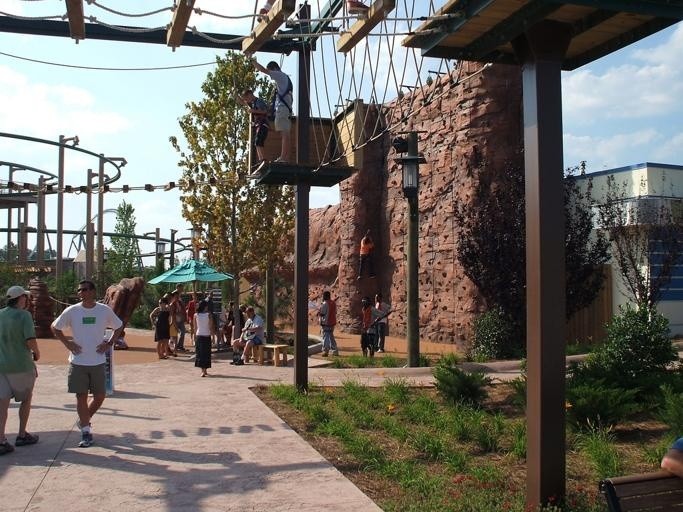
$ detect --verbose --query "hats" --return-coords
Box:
[6,286,31,299]
[245,306,254,313]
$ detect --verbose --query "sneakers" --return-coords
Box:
[15,431,38,446]
[332,350,338,356]
[230,359,245,365]
[0,441,14,456]
[170,346,185,352]
[322,352,328,357]
[78,419,94,447]
[201,372,205,377]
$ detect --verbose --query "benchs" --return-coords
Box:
[599,472,683,512]
[244,344,289,367]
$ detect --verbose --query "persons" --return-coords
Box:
[149,283,266,365]
[316,290,339,357]
[660,437,682,479]
[234,89,270,168]
[49,280,126,446]
[257,0,369,23]
[191,299,214,377]
[250,59,292,162]
[373,292,391,352]
[360,296,386,359]
[0,286,40,455]
[355,228,377,282]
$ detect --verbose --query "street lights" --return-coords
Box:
[393,131,425,366]
[155,241,166,297]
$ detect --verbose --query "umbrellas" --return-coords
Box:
[146,259,235,297]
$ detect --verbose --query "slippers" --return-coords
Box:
[159,356,169,359]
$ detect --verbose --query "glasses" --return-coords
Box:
[78,288,91,292]
[160,302,166,304]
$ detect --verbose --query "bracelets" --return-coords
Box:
[107,340,114,347]
